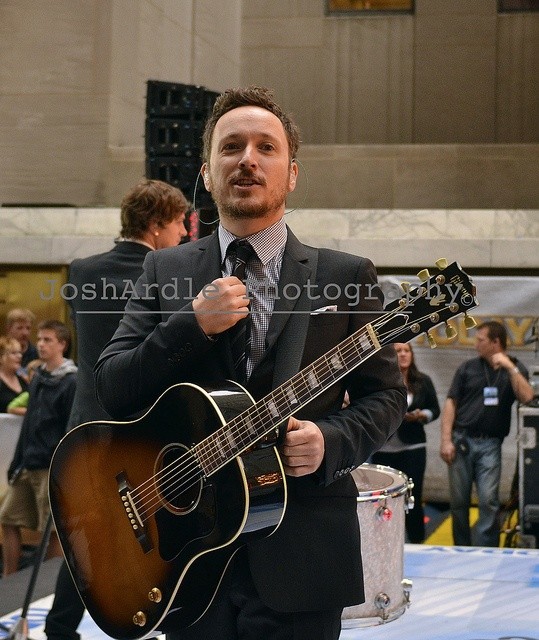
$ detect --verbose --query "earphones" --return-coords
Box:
[202,171,212,187]
[288,168,298,183]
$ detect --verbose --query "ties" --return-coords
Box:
[219,244,257,382]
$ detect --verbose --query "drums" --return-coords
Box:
[342,463,417,630]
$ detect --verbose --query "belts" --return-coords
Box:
[454,427,498,440]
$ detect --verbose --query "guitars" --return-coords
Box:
[48,258,480,638]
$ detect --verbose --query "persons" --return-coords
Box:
[93,87,407,640]
[7,307,39,365]
[64,179,191,640]
[0,337,30,409]
[439,321,534,547]
[369,342,441,544]
[0,318,79,575]
[7,359,45,415]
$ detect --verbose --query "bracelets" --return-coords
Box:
[509,367,518,376]
